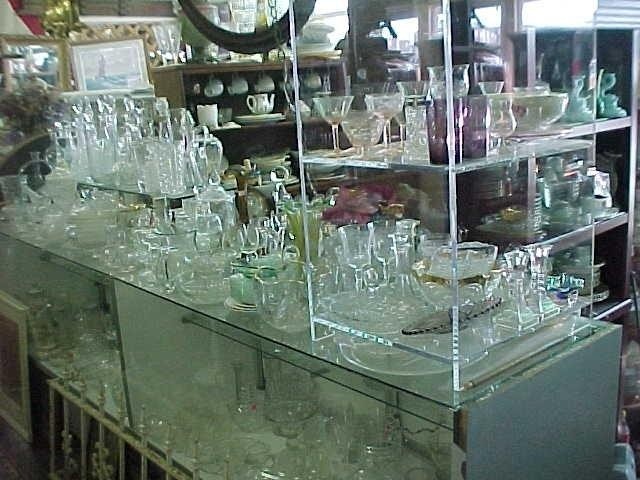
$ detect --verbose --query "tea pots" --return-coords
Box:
[245,92,276,115]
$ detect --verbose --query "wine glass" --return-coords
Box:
[326,213,437,325]
[149,20,187,70]
[400,62,517,158]
[500,246,561,331]
[323,91,402,154]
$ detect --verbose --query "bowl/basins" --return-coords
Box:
[296,40,331,53]
[520,84,569,136]
[579,196,612,219]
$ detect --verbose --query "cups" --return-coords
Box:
[197,103,217,128]
[407,99,488,170]
[286,190,337,260]
[2,94,278,297]
[28,317,434,480]
[219,108,230,129]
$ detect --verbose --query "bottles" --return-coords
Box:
[202,71,329,97]
[616,409,630,445]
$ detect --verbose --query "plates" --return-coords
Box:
[426,240,500,283]
[537,176,578,203]
[334,341,489,382]
[235,115,289,125]
[222,300,266,315]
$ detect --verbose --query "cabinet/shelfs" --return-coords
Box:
[140,1,640,392]
